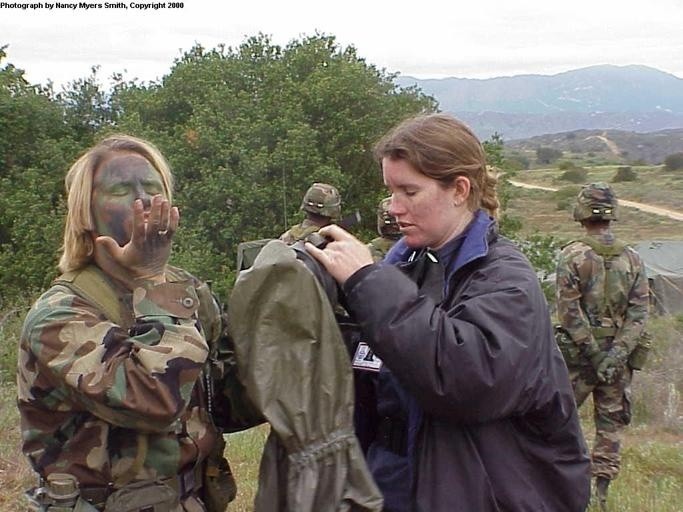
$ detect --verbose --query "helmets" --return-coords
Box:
[376,197,403,237]
[300,183,341,217]
[573,182,620,223]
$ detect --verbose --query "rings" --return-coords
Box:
[158,229,167,235]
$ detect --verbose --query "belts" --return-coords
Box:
[589,327,619,339]
[92,456,211,511]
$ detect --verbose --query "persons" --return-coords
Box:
[303,114,591,510]
[363,196,402,264]
[277,181,342,244]
[552,181,649,511]
[15,136,269,510]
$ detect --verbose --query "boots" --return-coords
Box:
[594,478,610,511]
